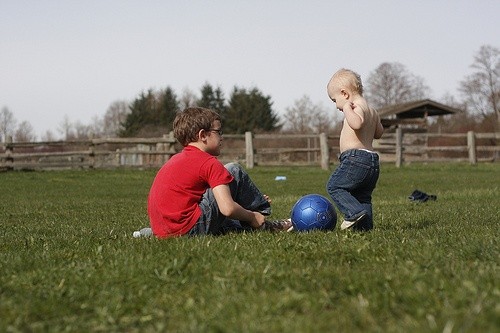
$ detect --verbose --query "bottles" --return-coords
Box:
[133,228,151,238]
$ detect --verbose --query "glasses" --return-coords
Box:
[206,129,223,135]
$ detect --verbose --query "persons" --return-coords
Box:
[327,68,384,232]
[148,107,295,240]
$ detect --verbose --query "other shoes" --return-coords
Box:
[270,218,295,235]
[339,211,366,231]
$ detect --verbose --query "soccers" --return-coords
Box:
[291,194,338,233]
